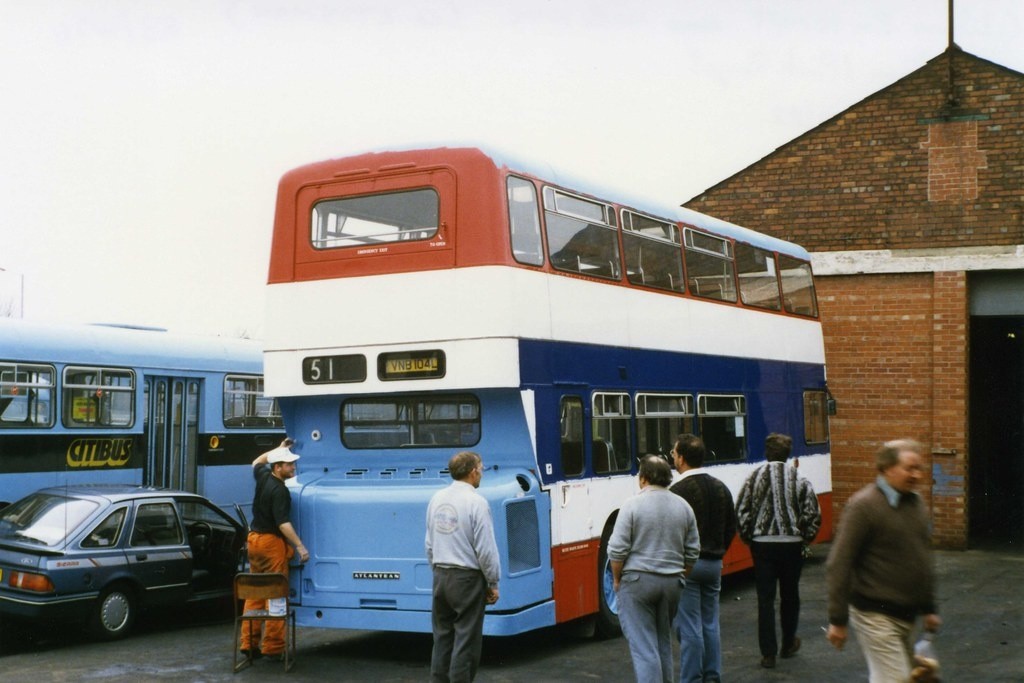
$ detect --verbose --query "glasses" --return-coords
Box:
[475,467,487,474]
[670,450,674,455]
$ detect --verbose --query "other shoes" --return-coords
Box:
[240,646,289,662]
[761,656,775,668]
[781,638,800,658]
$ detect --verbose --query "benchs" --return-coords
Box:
[353,428,618,473]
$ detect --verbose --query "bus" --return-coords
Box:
[261,147,838,642]
[0,319,287,575]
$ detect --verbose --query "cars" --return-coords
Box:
[0,483,251,641]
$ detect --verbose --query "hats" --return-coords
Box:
[267,447,300,464]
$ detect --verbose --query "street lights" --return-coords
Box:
[0,267,24,318]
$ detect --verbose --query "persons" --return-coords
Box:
[239,437,309,659]
[825,439,942,683]
[425,451,501,683]
[607,454,701,683]
[737,433,822,668]
[668,433,737,683]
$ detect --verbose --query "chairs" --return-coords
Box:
[516,249,813,315]
[144,510,178,545]
